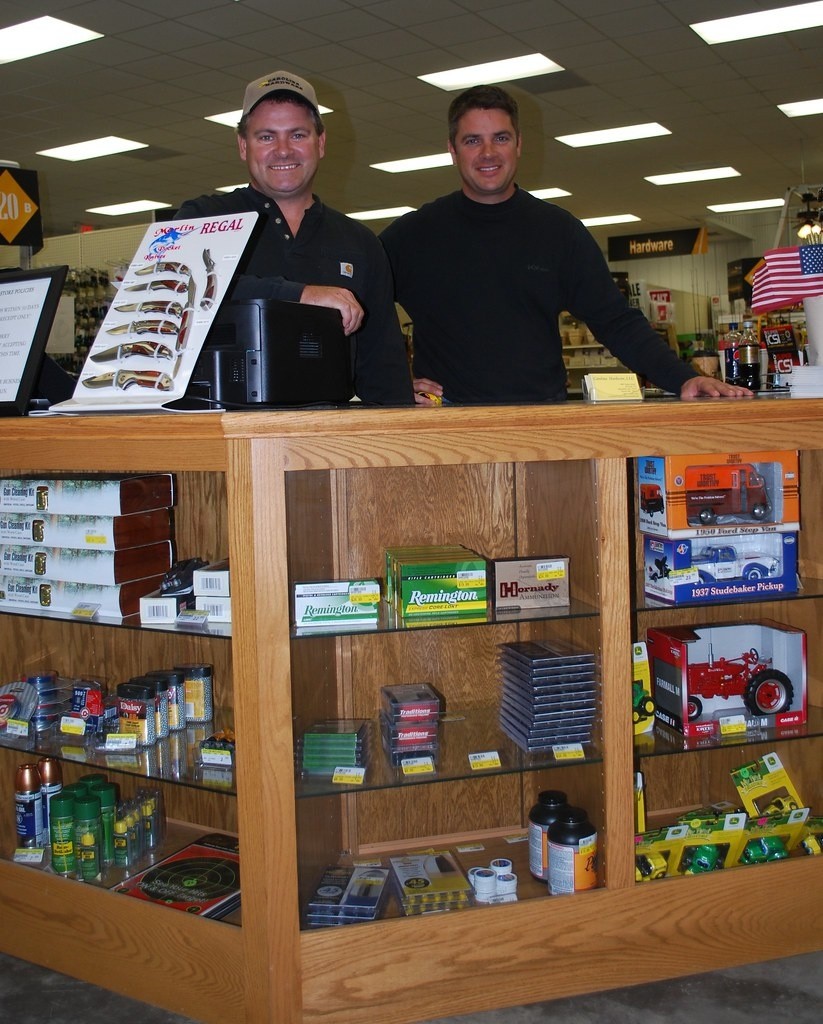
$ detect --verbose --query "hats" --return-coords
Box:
[241,72,320,120]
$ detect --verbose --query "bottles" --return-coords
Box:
[738,322,761,390]
[15,757,157,879]
[724,324,742,386]
[529,791,573,883]
[547,809,597,895]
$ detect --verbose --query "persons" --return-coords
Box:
[376,82,756,408]
[174,71,416,406]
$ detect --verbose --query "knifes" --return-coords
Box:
[114,301,183,319]
[106,320,179,335]
[82,369,174,391]
[90,341,172,363]
[135,262,192,276]
[173,275,197,379]
[123,280,188,293]
[199,249,217,310]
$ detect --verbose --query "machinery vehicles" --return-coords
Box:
[686,629,796,725]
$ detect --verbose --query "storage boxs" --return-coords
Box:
[294,544,570,627]
[644,620,807,738]
[301,640,597,774]
[633,449,800,537]
[641,534,799,605]
[0,475,231,627]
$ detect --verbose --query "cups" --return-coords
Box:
[716,349,768,389]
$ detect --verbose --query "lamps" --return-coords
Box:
[797,193,821,241]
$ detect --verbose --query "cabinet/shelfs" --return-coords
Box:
[0,400,823,1024]
[563,344,633,394]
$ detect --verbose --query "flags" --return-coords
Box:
[750,245,823,316]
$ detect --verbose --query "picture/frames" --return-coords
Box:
[639,321,679,390]
